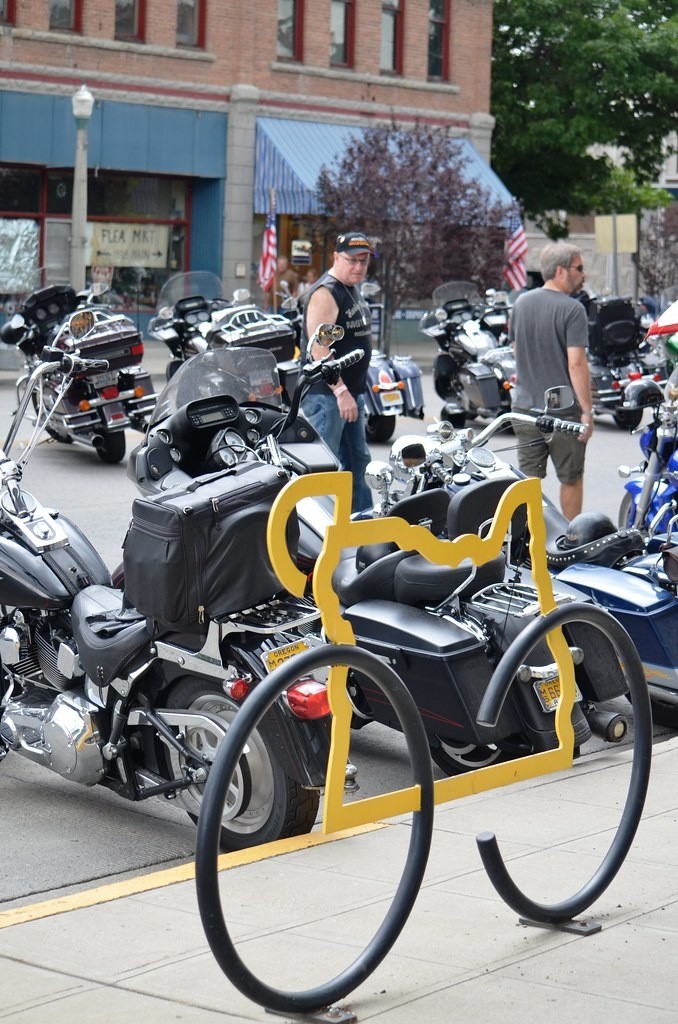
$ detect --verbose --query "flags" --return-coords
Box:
[505,200,529,291]
[257,196,277,291]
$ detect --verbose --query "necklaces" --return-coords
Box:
[344,283,367,326]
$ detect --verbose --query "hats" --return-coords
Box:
[336,232,375,256]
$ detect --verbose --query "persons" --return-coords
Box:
[301,232,375,514]
[264,256,298,317]
[297,268,318,298]
[508,242,595,522]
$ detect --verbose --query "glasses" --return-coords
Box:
[340,254,370,266]
[569,265,584,272]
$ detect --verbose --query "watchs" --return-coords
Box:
[584,408,595,418]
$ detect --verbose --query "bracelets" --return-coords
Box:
[333,384,347,395]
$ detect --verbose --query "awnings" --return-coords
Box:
[253,116,523,228]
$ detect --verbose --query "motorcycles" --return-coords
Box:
[0,283,158,462]
[349,413,678,741]
[567,290,672,429]
[147,271,421,441]
[0,352,330,849]
[123,347,594,776]
[619,405,678,556]
[419,282,517,429]
